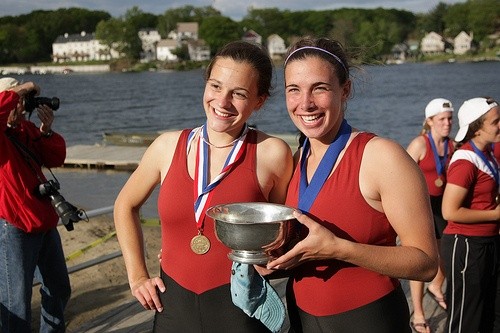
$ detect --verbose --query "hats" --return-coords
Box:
[0,77,20,94]
[231,261,286,333]
[423,98,454,126]
[454,97,498,142]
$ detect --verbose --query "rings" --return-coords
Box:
[45,117,48,120]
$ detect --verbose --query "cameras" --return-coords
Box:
[24,90,60,113]
[34,180,83,231]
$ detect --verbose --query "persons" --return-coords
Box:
[114,41,293,333]
[256,36,438,333]
[406,97,459,333]
[0,77,74,333]
[441,97,500,333]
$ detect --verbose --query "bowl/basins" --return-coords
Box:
[205,201,302,264]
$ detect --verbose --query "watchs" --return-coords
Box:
[41,129,52,138]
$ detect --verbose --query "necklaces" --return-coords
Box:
[200,131,250,148]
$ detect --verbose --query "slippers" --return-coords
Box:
[426,287,447,312]
[409,321,431,333]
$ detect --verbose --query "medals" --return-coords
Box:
[496,195,500,204]
[191,235,210,255]
[435,179,443,187]
